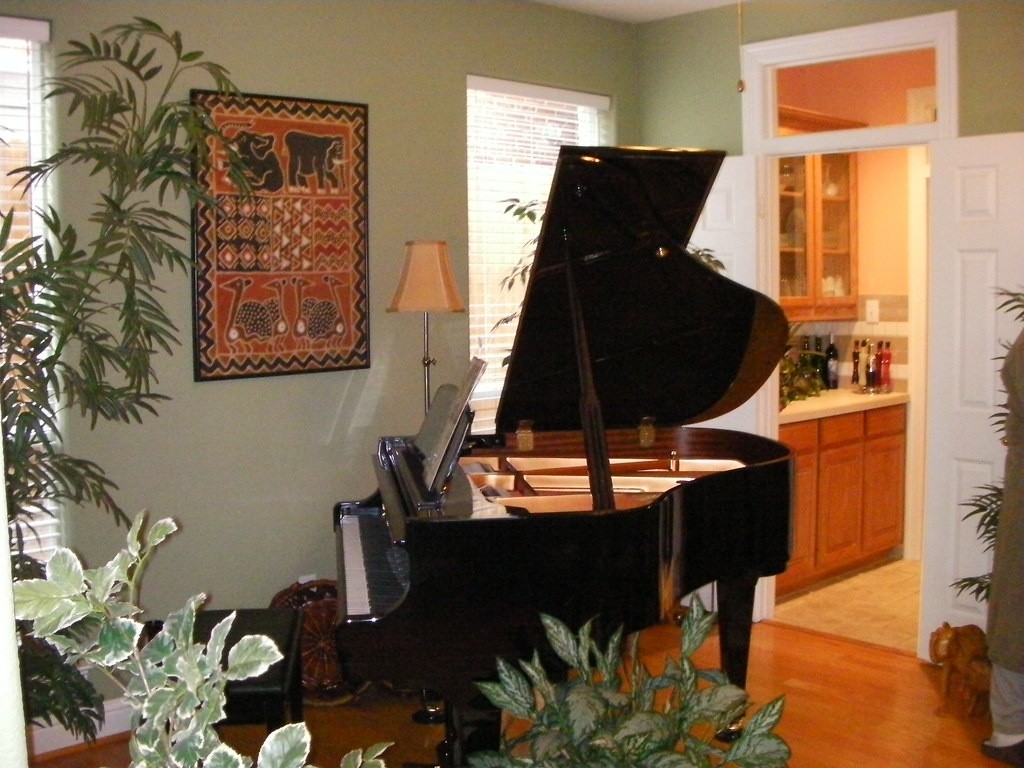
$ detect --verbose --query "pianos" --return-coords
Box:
[331,143,795,768]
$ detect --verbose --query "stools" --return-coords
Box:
[139,607,304,735]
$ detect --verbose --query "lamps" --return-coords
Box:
[385,239,465,413]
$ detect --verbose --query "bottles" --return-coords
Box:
[798,333,892,394]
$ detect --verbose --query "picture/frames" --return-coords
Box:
[188,87,372,382]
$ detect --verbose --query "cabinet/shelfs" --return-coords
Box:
[779,118,859,323]
[776,404,906,605]
